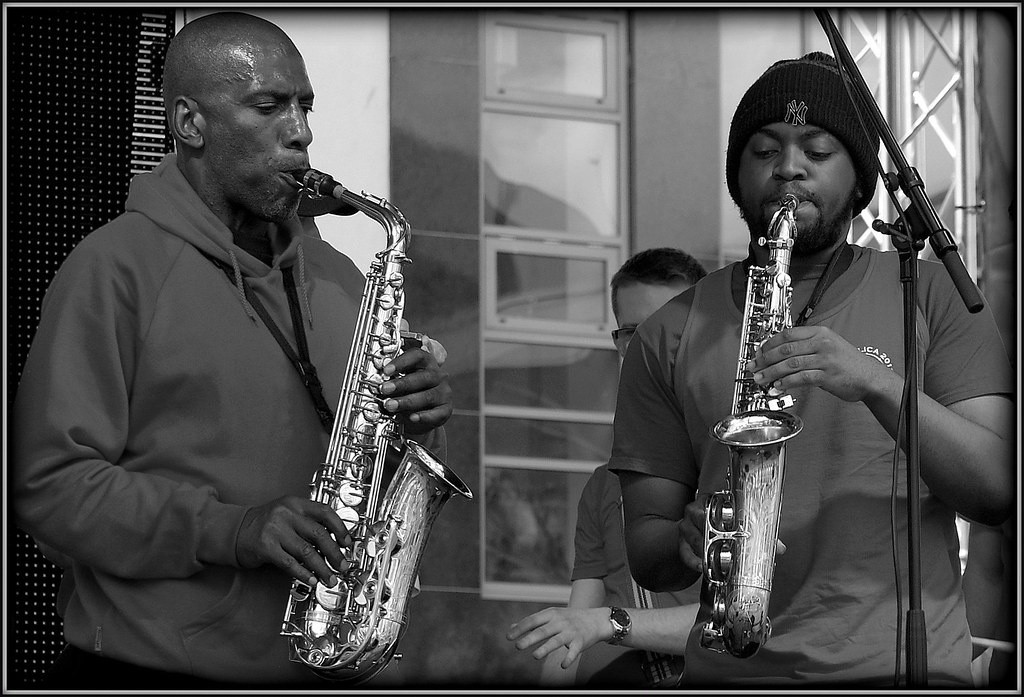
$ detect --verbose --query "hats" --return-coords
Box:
[726,52,880,220]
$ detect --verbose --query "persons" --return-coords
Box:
[10,13,456,690]
[608,52,1020,692]
[508,247,712,688]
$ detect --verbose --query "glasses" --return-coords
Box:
[611,327,636,358]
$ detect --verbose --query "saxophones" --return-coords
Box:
[699,190,806,656]
[281,162,473,682]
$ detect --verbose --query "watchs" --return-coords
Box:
[604,604,632,648]
